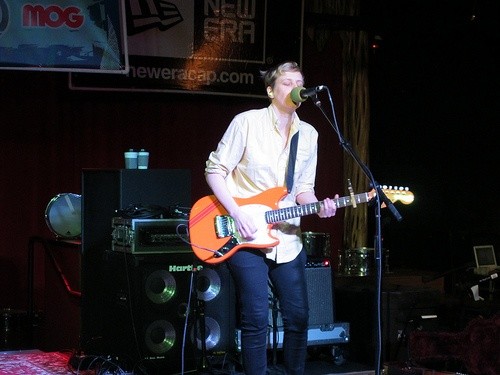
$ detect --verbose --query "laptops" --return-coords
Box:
[473,245,500,269]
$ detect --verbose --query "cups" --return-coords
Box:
[137,149,149,169]
[124,149,137,169]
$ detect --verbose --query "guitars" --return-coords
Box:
[188,185,415,264]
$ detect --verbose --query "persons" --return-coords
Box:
[204,61,339,375]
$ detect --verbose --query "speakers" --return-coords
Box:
[78,168,335,375]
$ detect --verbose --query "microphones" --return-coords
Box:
[290,85,326,103]
